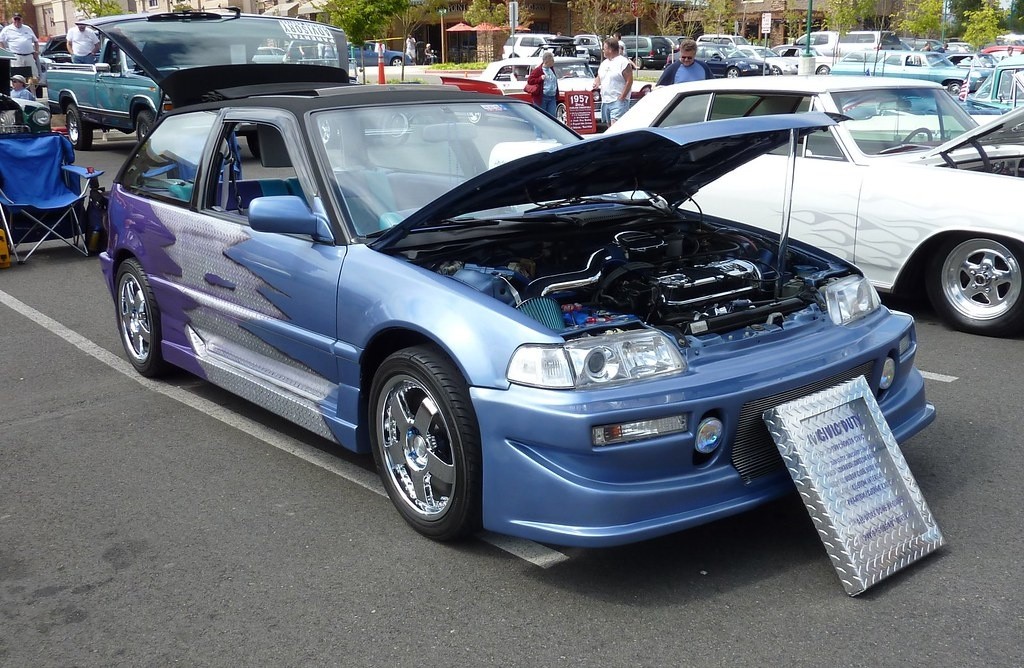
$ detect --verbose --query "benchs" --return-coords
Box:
[213,174,437,238]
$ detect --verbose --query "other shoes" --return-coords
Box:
[536,138,541,141]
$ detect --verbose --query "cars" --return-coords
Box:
[488,74,1023,334]
[72,12,937,550]
[0,6,1023,157]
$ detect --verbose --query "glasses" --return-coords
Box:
[11,79,16,83]
[681,56,693,60]
[13,18,21,22]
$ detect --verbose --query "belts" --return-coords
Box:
[14,53,34,56]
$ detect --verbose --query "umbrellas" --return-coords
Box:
[446,21,530,64]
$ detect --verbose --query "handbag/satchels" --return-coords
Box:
[523,84,542,96]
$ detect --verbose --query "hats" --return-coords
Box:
[426,44,430,48]
[12,13,23,19]
[78,16,85,21]
[10,75,26,84]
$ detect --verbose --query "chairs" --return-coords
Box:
[0,131,105,266]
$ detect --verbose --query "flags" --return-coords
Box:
[842,68,878,113]
[959,69,971,102]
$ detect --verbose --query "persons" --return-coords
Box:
[9,74,36,101]
[937,43,948,53]
[527,53,559,119]
[920,42,932,52]
[556,32,562,36]
[66,17,100,64]
[0,13,40,80]
[592,33,640,127]
[656,39,717,87]
[425,44,438,64]
[405,33,417,66]
[1000,46,1024,61]
